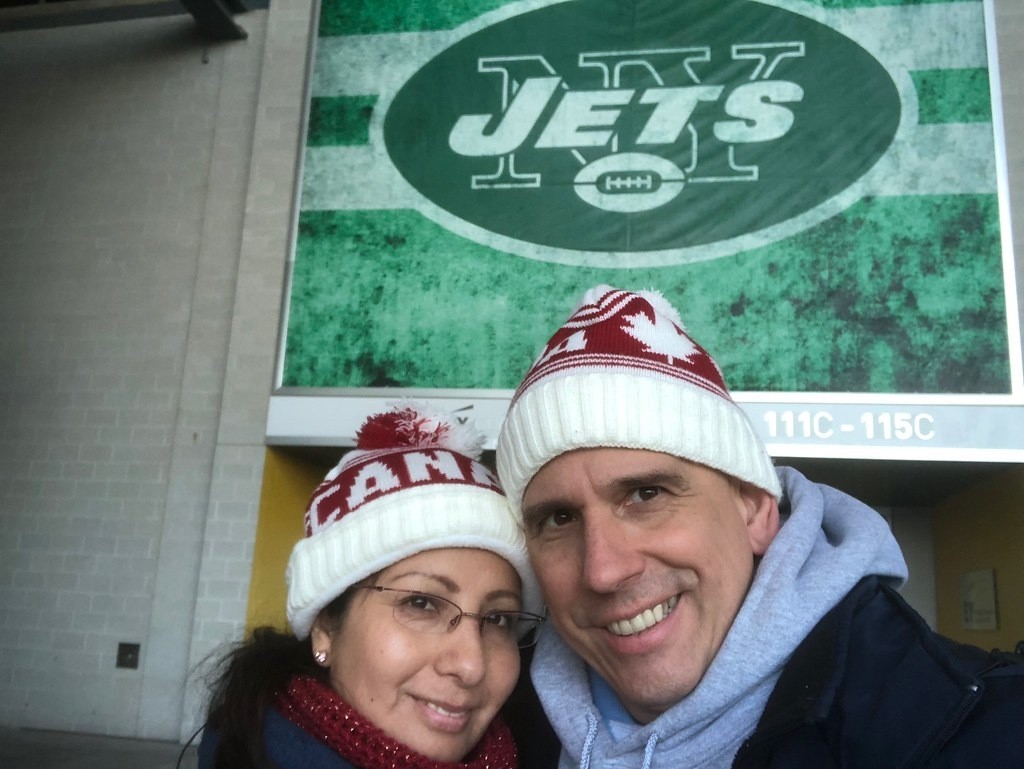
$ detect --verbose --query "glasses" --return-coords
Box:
[351,583,551,648]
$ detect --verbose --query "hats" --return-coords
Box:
[494,284,785,528]
[283,395,547,641]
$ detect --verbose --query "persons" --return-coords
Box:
[496,285,1024,769]
[197,404,548,769]
